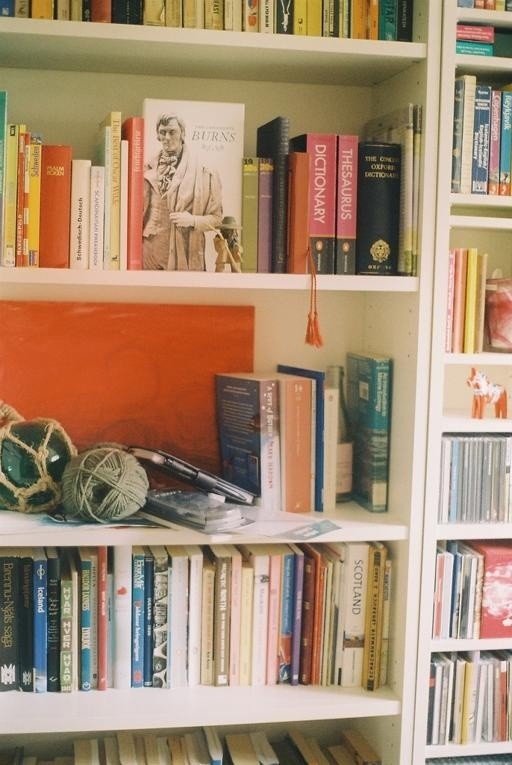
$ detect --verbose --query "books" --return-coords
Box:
[0,90,423,277]
[425,0,512,765]
[127,352,393,534]
[1,1,414,42]
[2,541,394,765]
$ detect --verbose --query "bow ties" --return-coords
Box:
[159,156,178,175]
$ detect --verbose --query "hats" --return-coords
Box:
[214,216,242,230]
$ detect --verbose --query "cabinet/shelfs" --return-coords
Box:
[1,1,512,765]
